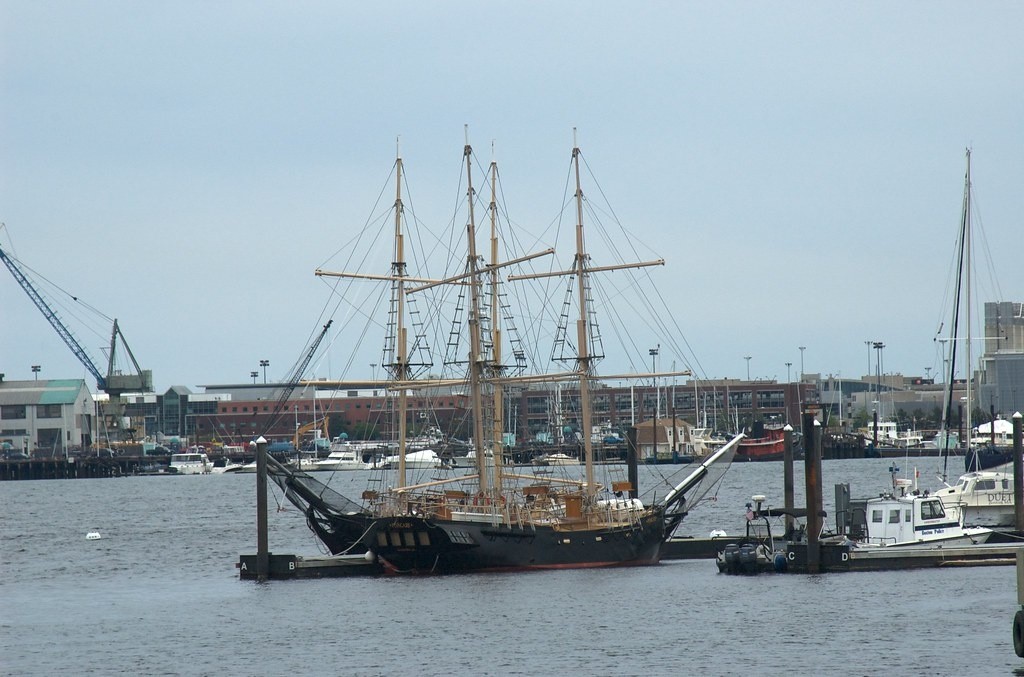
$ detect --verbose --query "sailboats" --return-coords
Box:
[240,121,748,573]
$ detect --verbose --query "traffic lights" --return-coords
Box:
[923,469,1018,527]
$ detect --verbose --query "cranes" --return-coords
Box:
[0,224,155,442]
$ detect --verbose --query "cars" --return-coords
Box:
[601,435,625,445]
[91,448,116,457]
[5,453,30,459]
[146,446,175,456]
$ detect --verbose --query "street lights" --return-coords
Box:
[865,340,875,416]
[31,364,42,379]
[785,361,793,384]
[648,347,658,386]
[798,346,807,380]
[260,359,269,384]
[873,341,884,402]
[744,356,753,389]
[250,371,260,383]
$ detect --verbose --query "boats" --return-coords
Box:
[852,474,994,550]
[448,440,496,468]
[963,414,1024,473]
[382,449,445,469]
[530,453,581,466]
[727,421,804,461]
[287,456,323,471]
[168,453,258,473]
[313,442,368,470]
